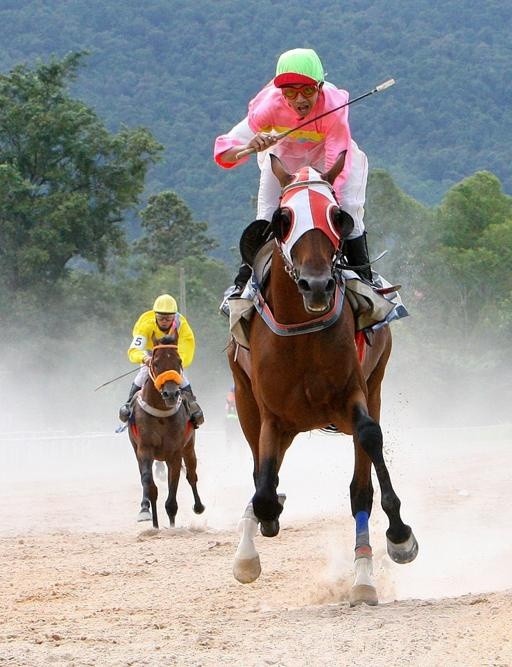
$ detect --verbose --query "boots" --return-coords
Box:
[220,259,253,318]
[343,231,378,294]
[180,385,204,425]
[119,383,141,423]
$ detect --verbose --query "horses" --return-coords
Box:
[128,327,205,529]
[226,149,418,608]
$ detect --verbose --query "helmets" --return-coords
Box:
[152,294,178,314]
[273,48,326,88]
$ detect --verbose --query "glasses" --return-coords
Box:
[155,313,175,321]
[279,85,322,101]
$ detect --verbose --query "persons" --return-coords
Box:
[213,46,373,317]
[119,295,204,425]
